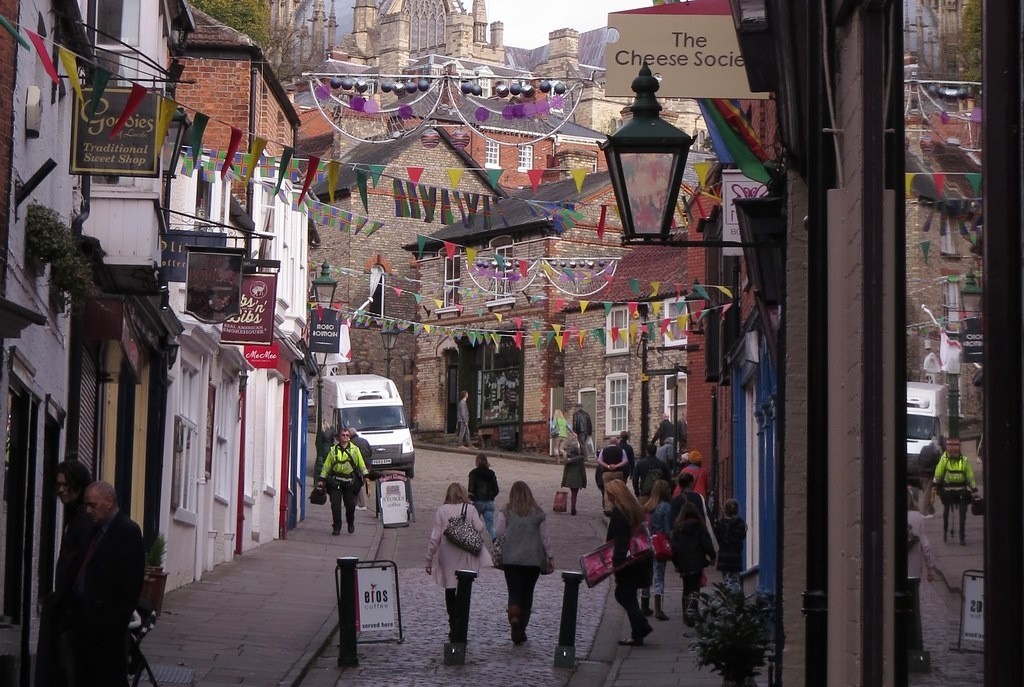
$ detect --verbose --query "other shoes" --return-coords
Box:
[469,446,478,450]
[617,591,699,646]
[571,509,577,516]
[457,445,468,449]
[511,618,522,643]
[331,505,368,536]
[921,513,966,547]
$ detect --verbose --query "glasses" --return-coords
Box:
[945,443,961,447]
[341,433,350,437]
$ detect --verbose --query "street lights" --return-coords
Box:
[314,351,327,488]
[944,302,963,438]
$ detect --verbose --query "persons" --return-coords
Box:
[495,481,553,645]
[315,417,335,464]
[457,390,478,449]
[423,482,493,643]
[318,428,370,535]
[347,428,372,511]
[596,410,748,625]
[468,453,499,541]
[550,410,576,465]
[603,479,656,646]
[573,404,592,462]
[560,448,586,515]
[906,430,979,655]
[50,460,146,687]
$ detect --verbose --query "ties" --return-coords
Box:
[72,529,104,586]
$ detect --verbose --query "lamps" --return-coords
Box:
[47,7,198,181]
[961,269,984,318]
[595,60,787,248]
[684,277,709,333]
[309,259,339,310]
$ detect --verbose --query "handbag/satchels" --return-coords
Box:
[551,417,559,438]
[443,501,483,555]
[586,435,595,453]
[651,530,673,561]
[906,524,920,550]
[929,487,937,505]
[553,486,567,512]
[309,485,326,505]
[971,495,985,516]
[351,474,363,494]
[706,516,720,567]
[666,446,679,469]
[491,509,507,570]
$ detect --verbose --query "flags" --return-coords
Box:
[699,99,777,184]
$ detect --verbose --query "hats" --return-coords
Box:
[664,436,703,464]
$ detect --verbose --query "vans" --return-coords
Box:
[907,381,949,487]
[315,373,415,479]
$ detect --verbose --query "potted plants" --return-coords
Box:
[25,200,97,316]
[146,533,169,617]
[683,572,777,687]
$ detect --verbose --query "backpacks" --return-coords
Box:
[640,458,663,496]
